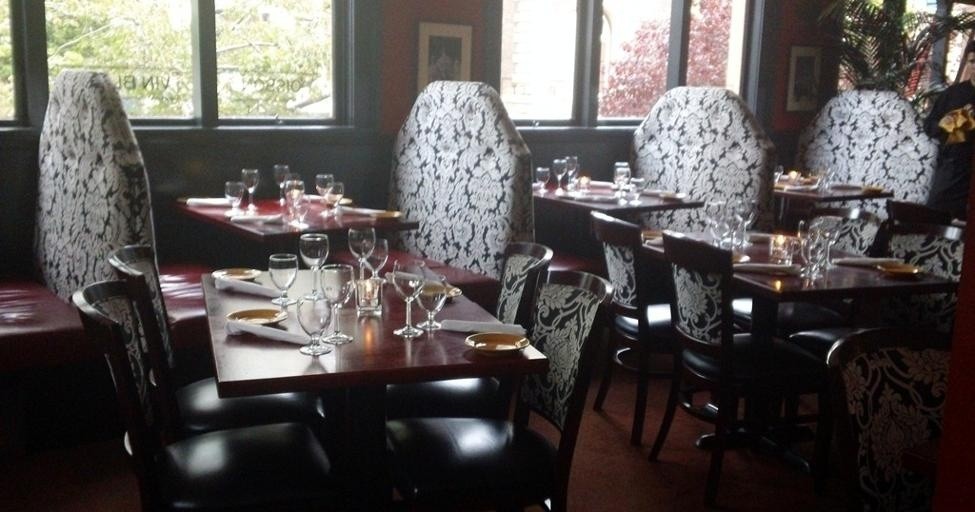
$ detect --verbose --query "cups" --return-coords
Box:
[768,234,794,268]
[774,165,784,186]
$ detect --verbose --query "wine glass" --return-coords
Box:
[798,215,845,281]
[702,198,760,265]
[814,165,835,198]
[535,155,645,206]
[224,162,347,228]
[266,225,448,356]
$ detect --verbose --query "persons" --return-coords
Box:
[924,37,975,220]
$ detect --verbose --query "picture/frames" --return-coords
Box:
[784,43,825,114]
[416,19,475,98]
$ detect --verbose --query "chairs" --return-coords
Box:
[590,83,968,512]
[72,280,345,512]
[323,80,604,313]
[385,270,614,512]
[385,241,554,420]
[108,244,328,444]
[0,68,240,473]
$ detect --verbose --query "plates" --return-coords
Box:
[876,263,923,280]
[659,192,688,203]
[370,210,402,218]
[327,195,351,206]
[224,308,286,328]
[461,330,530,357]
[418,283,466,301]
[210,266,262,282]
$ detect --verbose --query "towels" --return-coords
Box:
[300,193,324,203]
[440,318,526,340]
[230,213,283,226]
[531,181,544,191]
[575,195,618,203]
[225,318,311,347]
[385,271,427,288]
[186,195,241,208]
[330,206,387,217]
[212,274,282,300]
